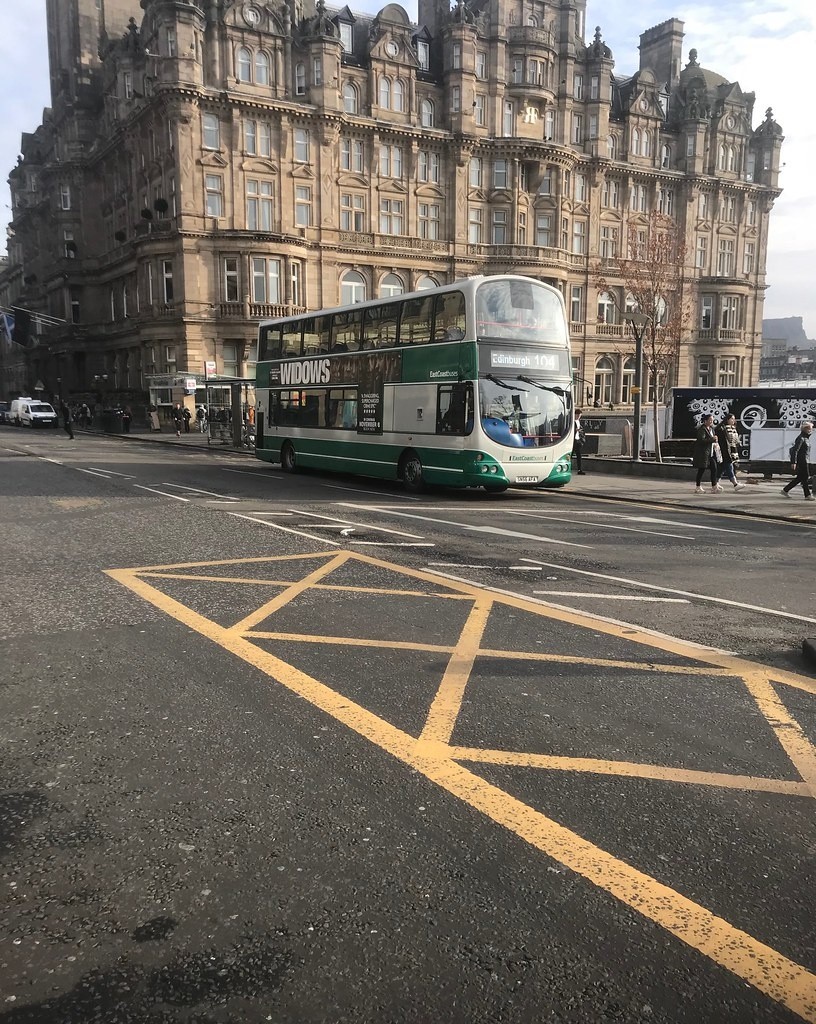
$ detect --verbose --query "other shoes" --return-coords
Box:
[734,484,746,491]
[711,486,721,494]
[717,483,723,489]
[805,495,815,501]
[780,489,792,498]
[695,486,706,494]
[578,470,585,475]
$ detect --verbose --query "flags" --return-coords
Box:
[4,309,30,347]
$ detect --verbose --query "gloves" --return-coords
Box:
[175,418,177,420]
[183,418,185,420]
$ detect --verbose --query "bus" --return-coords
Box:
[255,275,594,492]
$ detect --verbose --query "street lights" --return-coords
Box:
[57,377,62,401]
[620,312,652,462]
[95,375,108,430]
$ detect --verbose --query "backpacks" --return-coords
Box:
[197,408,204,418]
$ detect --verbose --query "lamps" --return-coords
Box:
[145,47,162,57]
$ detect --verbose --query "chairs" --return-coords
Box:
[278,399,305,425]
[265,340,464,357]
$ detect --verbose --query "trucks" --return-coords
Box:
[9,400,58,428]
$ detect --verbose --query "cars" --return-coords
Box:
[0,401,11,419]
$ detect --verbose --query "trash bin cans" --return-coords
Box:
[102,408,125,433]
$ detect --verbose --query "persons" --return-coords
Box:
[72,403,90,429]
[62,402,75,440]
[692,415,723,494]
[183,406,192,433]
[197,404,207,434]
[113,404,132,433]
[780,421,816,501]
[594,399,600,408]
[147,402,156,417]
[609,402,614,408]
[171,403,185,436]
[571,409,586,475]
[716,413,746,491]
[522,318,537,333]
[210,402,232,446]
